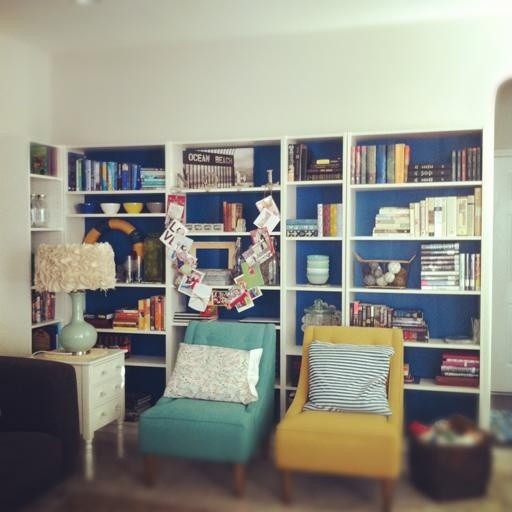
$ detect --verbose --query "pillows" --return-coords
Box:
[302,340,395,418]
[163,343,263,406]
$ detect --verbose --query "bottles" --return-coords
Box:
[30,192,49,228]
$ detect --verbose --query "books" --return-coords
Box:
[286,203,344,237]
[351,144,482,184]
[84,295,165,331]
[373,187,481,237]
[222,201,243,233]
[173,311,219,324]
[437,349,480,388]
[70,158,166,190]
[95,332,133,358]
[288,143,343,181]
[404,363,414,383]
[350,301,430,343]
[186,147,254,189]
[420,242,481,291]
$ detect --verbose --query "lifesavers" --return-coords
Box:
[82,219,144,280]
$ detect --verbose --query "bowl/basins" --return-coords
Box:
[146,201,165,213]
[306,255,331,285]
[77,202,98,214]
[123,202,144,214]
[99,202,121,214]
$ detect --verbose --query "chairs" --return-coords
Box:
[0,356,84,512]
[139,319,278,498]
[271,325,406,511]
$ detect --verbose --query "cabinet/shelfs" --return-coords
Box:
[347,127,485,427]
[167,135,284,422]
[29,142,66,354]
[66,143,167,370]
[284,133,347,419]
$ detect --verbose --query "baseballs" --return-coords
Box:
[362,262,408,286]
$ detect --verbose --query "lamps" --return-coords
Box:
[33,242,117,355]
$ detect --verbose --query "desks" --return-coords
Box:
[30,349,127,480]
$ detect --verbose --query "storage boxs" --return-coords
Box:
[409,430,492,502]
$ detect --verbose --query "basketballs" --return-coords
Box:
[32,327,50,351]
